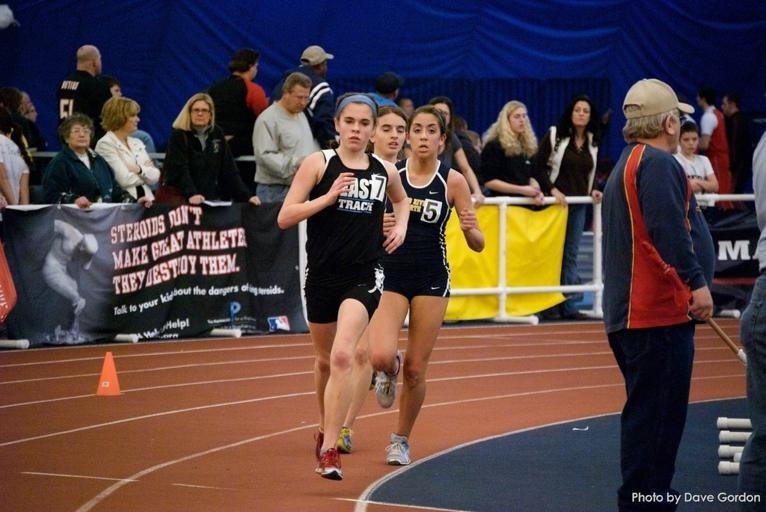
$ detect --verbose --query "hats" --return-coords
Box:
[300,46,335,68]
[622,78,696,119]
[374,72,404,94]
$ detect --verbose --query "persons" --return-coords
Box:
[0,42,765,326]
[365,104,486,467]
[275,90,411,483]
[337,102,408,455]
[734,121,766,512]
[39,218,100,344]
[597,76,720,511]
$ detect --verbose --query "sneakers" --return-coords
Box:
[313,349,411,481]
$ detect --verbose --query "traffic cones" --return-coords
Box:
[95,348,124,398]
